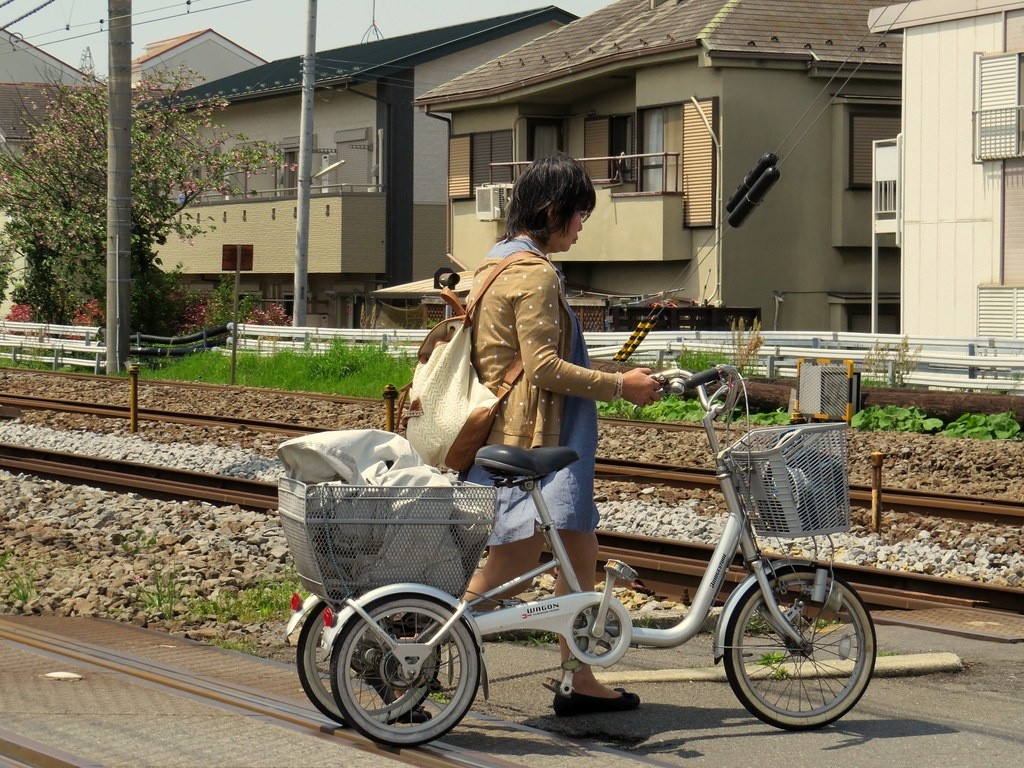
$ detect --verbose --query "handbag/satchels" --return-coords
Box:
[757,429,846,532]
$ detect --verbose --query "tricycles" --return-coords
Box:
[275,365,878,749]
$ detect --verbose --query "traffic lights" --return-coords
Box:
[434,268,461,289]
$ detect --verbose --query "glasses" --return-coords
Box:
[578,210,590,223]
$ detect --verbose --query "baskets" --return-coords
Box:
[278,476,497,613]
[731,423,850,537]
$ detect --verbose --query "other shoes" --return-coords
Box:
[553,687,640,715]
[393,706,431,722]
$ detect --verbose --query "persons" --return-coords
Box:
[371,150,662,727]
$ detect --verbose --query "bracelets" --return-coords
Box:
[615,370,625,400]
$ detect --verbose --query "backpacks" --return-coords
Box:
[407,251,538,473]
